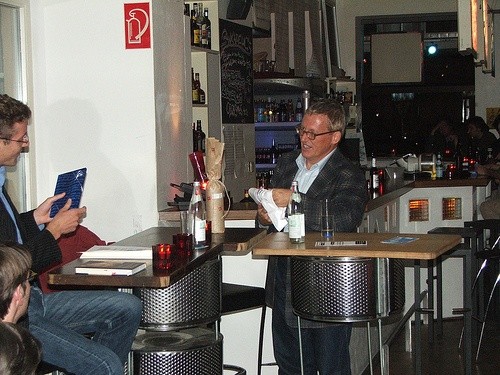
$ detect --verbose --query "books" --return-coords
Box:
[75,260,146,277]
[78,246,155,260]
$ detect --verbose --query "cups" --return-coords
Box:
[153,262,174,276]
[180,210,195,248]
[175,251,192,266]
[152,243,175,262]
[320,199,335,238]
[173,233,192,251]
[379,168,384,195]
[206,220,212,242]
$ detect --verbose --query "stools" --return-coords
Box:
[413,219,500,375]
[215,281,265,375]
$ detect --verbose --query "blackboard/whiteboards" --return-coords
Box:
[217,18,255,124]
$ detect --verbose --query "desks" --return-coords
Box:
[252,231,461,375]
[117,226,267,251]
[47,240,222,375]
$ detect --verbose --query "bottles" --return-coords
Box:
[192,119,205,153]
[370,152,379,191]
[244,169,273,199]
[436,143,493,180]
[254,134,301,164]
[192,68,205,104]
[185,3,211,50]
[188,181,210,249]
[330,87,357,127]
[287,181,306,244]
[253,89,311,124]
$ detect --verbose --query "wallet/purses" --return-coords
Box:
[50,167,87,217]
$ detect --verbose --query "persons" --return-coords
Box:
[0,93,144,375]
[435,117,500,219]
[249,98,370,375]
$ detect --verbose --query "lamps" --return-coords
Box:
[426,44,440,56]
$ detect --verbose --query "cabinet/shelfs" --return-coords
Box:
[149,0,343,205]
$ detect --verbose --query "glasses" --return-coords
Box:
[295,124,341,139]
[0,133,30,143]
[17,269,38,286]
